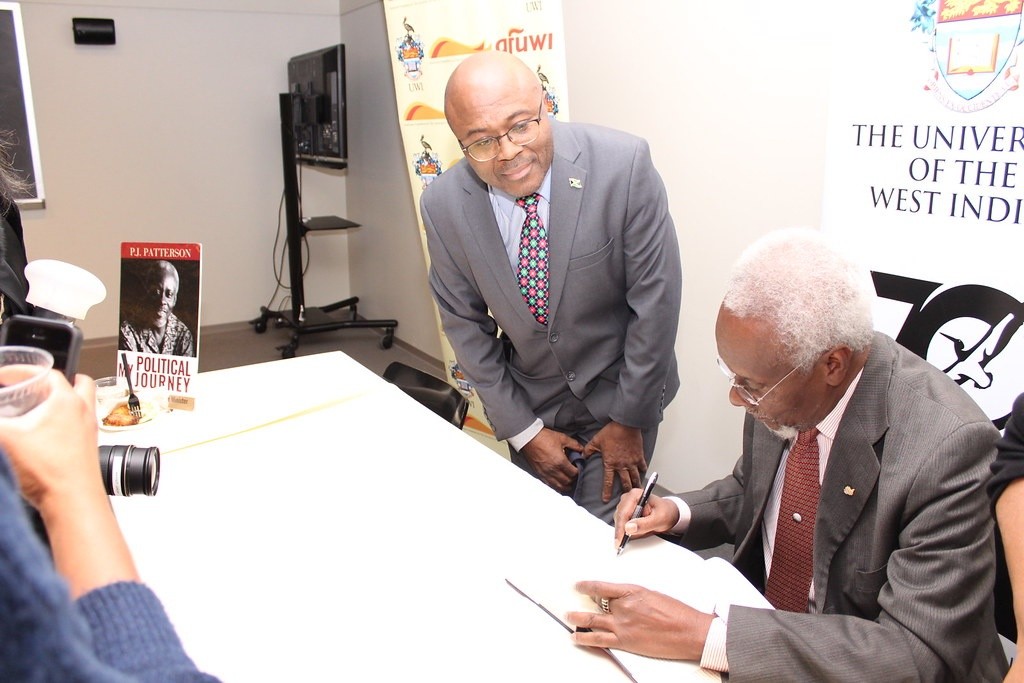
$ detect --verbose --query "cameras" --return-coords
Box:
[93,446,161,498]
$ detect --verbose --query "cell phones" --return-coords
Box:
[2,314,81,387]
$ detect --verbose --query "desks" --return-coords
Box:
[83,350,635,683]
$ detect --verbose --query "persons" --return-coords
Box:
[420,50,683,527]
[0,365,220,683]
[984,391,1024,683]
[562,225,1010,683]
[119,260,195,357]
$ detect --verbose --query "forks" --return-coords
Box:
[122,353,142,419]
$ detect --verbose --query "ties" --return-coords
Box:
[514,193,553,326]
[762,426,822,613]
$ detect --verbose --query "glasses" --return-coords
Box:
[457,88,543,162]
[715,350,821,406]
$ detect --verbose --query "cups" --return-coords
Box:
[95,377,128,407]
[0,345,55,418]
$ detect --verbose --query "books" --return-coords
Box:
[116,242,203,396]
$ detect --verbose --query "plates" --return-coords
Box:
[95,401,157,431]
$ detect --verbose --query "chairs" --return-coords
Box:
[383,362,469,430]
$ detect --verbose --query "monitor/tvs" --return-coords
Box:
[286,43,347,170]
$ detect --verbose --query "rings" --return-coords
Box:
[601,597,610,613]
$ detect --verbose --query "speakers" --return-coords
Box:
[73,18,116,45]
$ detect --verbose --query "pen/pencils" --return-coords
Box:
[616,471,659,556]
